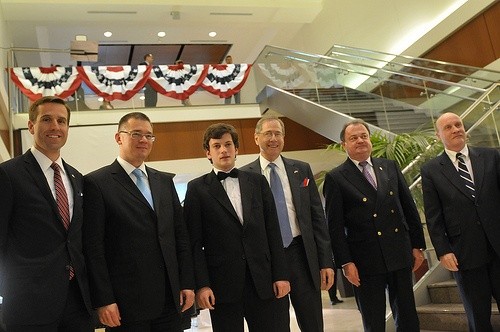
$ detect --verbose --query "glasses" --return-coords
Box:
[120,130,156,143]
[256,132,283,137]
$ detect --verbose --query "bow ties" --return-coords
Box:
[217,168,238,181]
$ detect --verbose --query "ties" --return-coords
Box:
[51,162,75,281]
[267,163,294,249]
[456,153,476,200]
[359,161,377,190]
[132,169,154,210]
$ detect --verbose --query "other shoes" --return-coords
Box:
[331,299,343,305]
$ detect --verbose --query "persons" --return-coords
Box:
[139,54,157,108]
[176,60,191,106]
[326,257,343,305]
[421,112,500,332]
[184,123,291,332]
[323,122,427,332]
[225,56,240,104]
[83,113,195,332]
[238,116,334,332]
[0,96,83,332]
